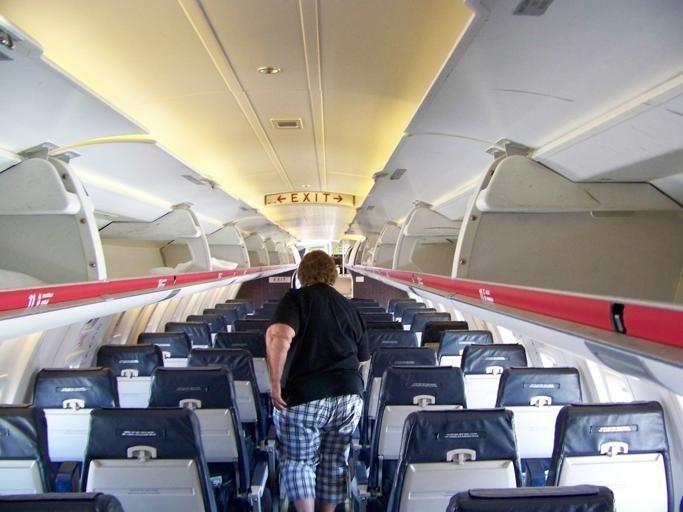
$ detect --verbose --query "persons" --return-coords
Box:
[265,250,372,512]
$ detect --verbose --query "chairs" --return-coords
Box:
[0,296,282,512]
[347,293,677,511]
